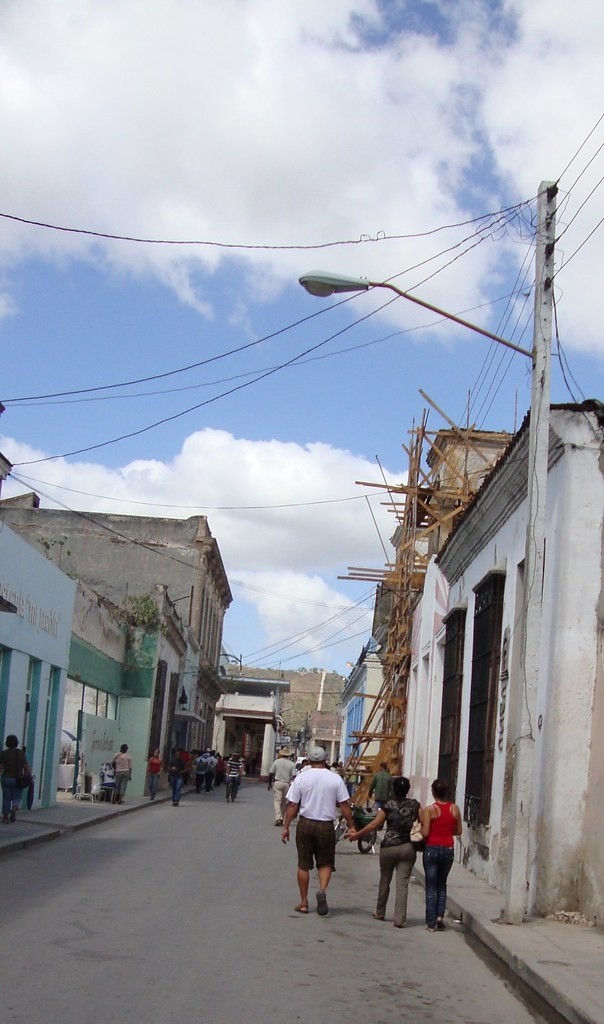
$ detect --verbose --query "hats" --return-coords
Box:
[309,746,325,761]
[279,747,292,756]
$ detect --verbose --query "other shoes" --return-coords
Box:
[394,924,403,928]
[171,801,179,805]
[274,820,283,826]
[373,912,384,920]
[10,809,15,822]
[2,815,9,823]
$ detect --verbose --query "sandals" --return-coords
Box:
[437,916,445,929]
[426,926,435,932]
[316,891,328,916]
[295,904,308,913]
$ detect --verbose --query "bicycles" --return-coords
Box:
[224,773,240,803]
[332,803,382,853]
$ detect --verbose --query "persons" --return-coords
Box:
[110,744,132,805]
[0,735,29,824]
[347,779,423,928]
[420,778,463,931]
[146,748,165,800]
[365,761,393,812]
[292,759,357,794]
[168,748,248,807]
[267,747,296,825]
[281,747,356,916]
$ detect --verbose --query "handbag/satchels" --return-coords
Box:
[112,762,116,768]
[27,784,34,811]
[18,775,30,788]
[410,799,423,842]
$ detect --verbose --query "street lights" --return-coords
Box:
[298,273,551,923]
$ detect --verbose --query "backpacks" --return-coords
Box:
[196,758,205,772]
